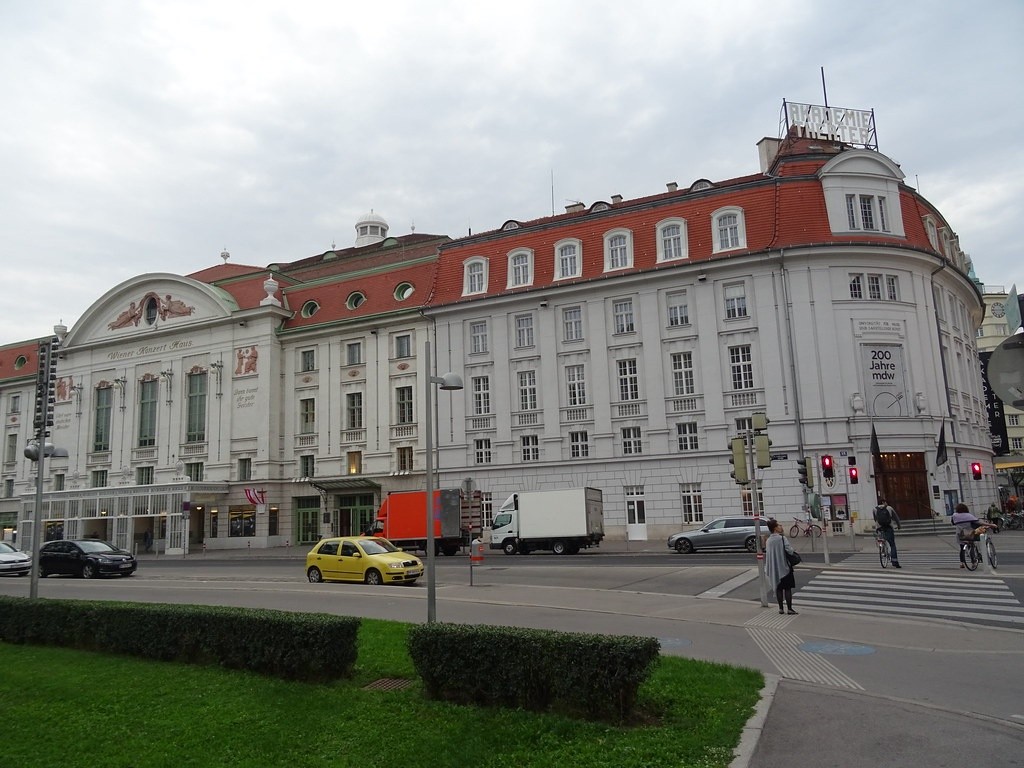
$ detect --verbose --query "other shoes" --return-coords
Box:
[960,563,965,568]
[787,610,799,614]
[779,609,784,614]
[892,561,901,568]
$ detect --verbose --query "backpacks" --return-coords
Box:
[875,505,891,526]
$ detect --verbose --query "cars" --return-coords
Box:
[0,542,32,578]
[305,536,425,587]
[668,515,776,554]
[38,538,137,580]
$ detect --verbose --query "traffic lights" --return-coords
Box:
[848,456,856,465]
[849,468,858,484]
[752,413,770,431]
[797,457,813,488]
[727,437,748,486]
[821,456,835,478]
[971,462,982,480]
[754,434,772,469]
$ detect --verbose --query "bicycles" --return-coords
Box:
[877,526,899,568]
[962,526,998,571]
[980,512,1024,531]
[789,517,822,538]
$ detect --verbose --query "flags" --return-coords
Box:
[871,422,886,477]
[935,419,948,466]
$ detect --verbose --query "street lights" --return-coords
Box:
[24,337,59,598]
[425,340,463,623]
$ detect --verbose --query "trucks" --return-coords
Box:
[489,487,606,556]
[358,487,484,557]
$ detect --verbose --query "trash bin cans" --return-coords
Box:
[471,539,484,566]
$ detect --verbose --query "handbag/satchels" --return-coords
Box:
[782,535,802,567]
[960,527,981,541]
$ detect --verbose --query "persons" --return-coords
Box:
[951,504,997,568]
[988,503,1003,533]
[144,527,152,552]
[764,520,799,615]
[871,497,902,568]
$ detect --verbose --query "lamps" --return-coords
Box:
[159,371,173,405]
[210,363,223,398]
[102,512,106,515]
[69,386,83,416]
[112,379,127,412]
[197,506,202,510]
